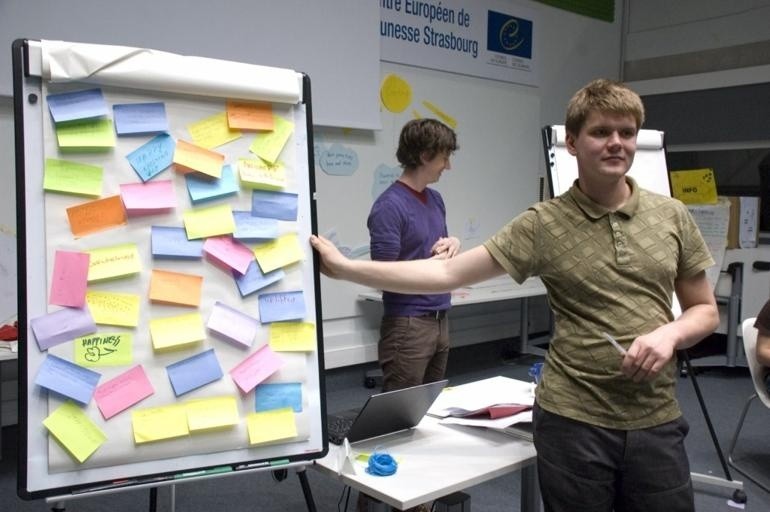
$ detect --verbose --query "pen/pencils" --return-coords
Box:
[603,331,626,356]
[71,459,291,494]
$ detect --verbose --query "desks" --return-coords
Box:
[356,277,553,356]
[308,375,537,512]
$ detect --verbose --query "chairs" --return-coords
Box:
[729,317,770,493]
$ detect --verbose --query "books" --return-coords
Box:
[486,422,534,441]
[444,393,535,418]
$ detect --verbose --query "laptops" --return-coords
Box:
[326,378,450,446]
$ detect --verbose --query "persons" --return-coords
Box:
[364,119,463,394]
[752,297,769,399]
[308,76,721,512]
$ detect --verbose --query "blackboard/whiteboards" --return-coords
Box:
[11,38,328,503]
[542,126,684,319]
[0,60,546,361]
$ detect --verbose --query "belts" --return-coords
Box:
[429,310,446,320]
